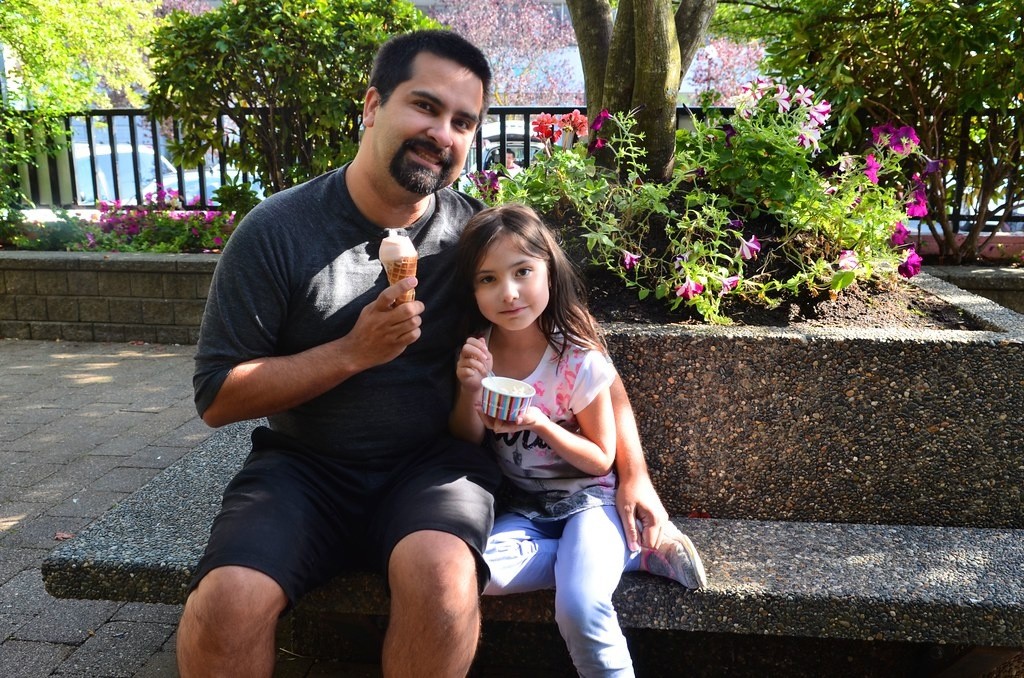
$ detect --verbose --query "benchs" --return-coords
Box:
[42,417,1024,678]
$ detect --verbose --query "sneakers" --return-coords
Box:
[636,519,706,589]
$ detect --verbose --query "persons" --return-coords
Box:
[175,29,669,678]
[507,149,521,178]
[448,203,706,678]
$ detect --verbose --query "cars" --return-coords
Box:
[70,142,267,210]
[470,119,552,172]
